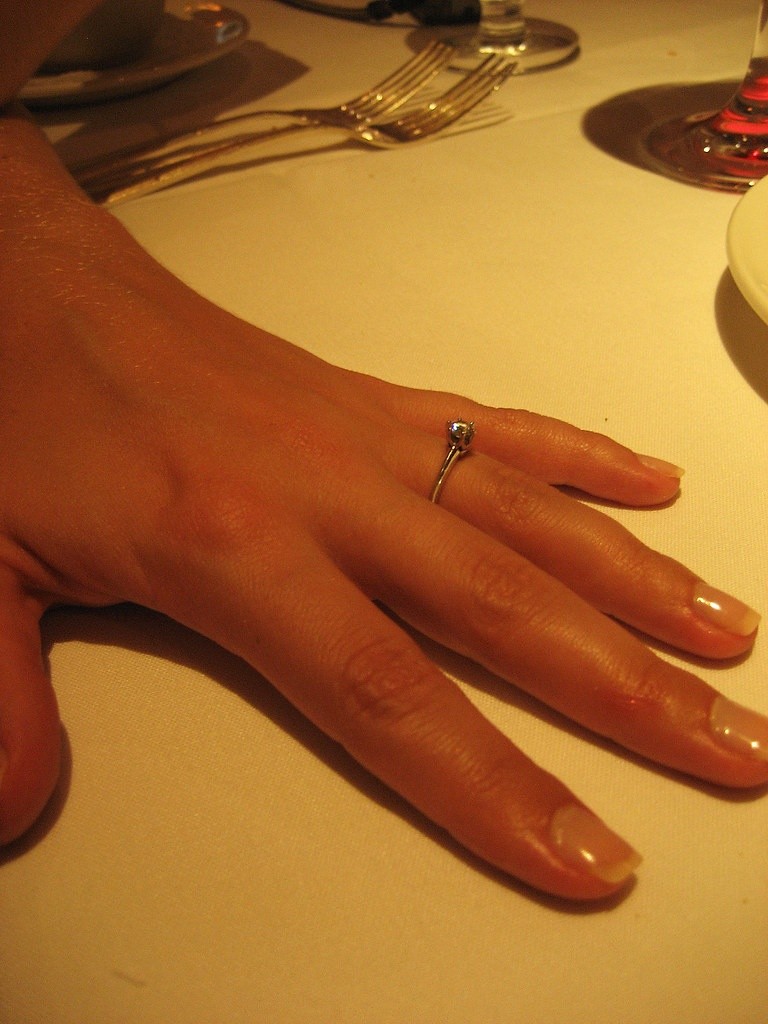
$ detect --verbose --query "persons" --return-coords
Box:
[0,0,768,902]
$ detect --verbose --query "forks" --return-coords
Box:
[58,37,467,179]
[81,50,520,215]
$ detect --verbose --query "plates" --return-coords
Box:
[726,173,768,332]
[22,2,247,109]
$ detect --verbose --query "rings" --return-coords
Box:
[428,415,477,508]
